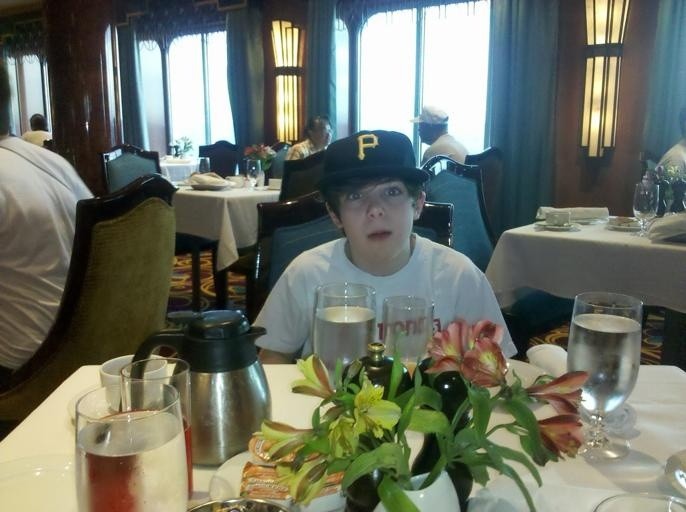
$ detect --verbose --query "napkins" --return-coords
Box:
[535,206,610,224]
[524,338,567,377]
[646,210,686,241]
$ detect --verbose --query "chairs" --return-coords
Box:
[244,191,454,330]
[100,142,161,197]
[2,171,181,441]
[278,144,330,201]
[198,138,242,177]
[464,148,507,226]
[419,156,575,337]
[42,137,80,171]
[0,363,686,510]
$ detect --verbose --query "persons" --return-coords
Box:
[0,59,99,390]
[286,112,334,162]
[20,114,54,148]
[244,131,519,365]
[654,104,686,185]
[411,106,470,166]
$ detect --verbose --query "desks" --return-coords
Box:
[173,172,285,309]
[486,214,686,373]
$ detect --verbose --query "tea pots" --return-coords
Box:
[120,315,275,469]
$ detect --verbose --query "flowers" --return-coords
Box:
[240,318,593,510]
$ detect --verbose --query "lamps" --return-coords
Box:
[266,16,306,146]
[578,0,635,190]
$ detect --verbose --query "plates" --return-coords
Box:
[473,356,547,399]
[543,222,582,231]
[190,181,234,189]
[608,224,642,231]
[210,451,348,511]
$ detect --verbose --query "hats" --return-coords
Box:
[409,104,450,126]
[314,128,431,194]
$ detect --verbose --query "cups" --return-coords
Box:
[268,179,281,190]
[592,491,686,512]
[382,296,437,370]
[101,352,167,413]
[228,176,245,188]
[121,357,193,500]
[313,282,377,383]
[545,212,571,225]
[74,382,189,512]
[187,498,291,512]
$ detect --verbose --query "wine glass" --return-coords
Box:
[246,159,262,192]
[564,292,643,463]
[631,183,660,238]
